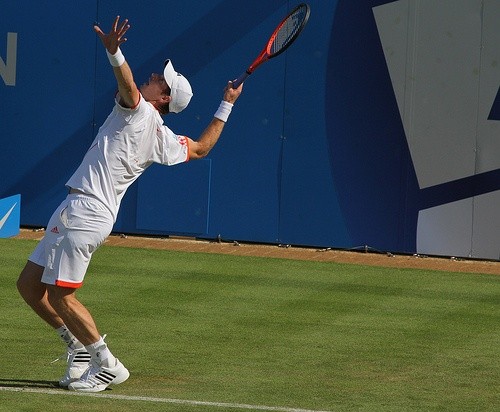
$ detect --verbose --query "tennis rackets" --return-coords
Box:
[232,3,310,90]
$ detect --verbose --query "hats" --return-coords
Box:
[162,58,194,114]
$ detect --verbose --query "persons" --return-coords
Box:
[16,14,244,393]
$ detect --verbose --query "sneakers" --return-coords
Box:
[59,345,91,387]
[68,359,130,392]
